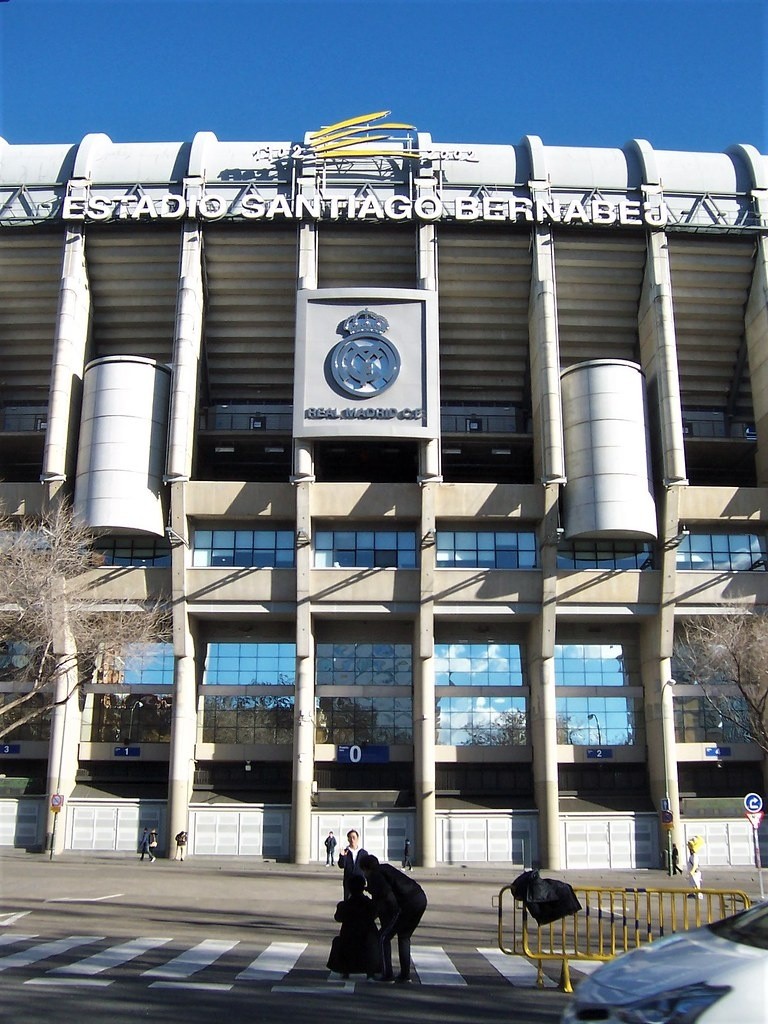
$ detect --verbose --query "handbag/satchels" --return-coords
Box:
[149,841,157,847]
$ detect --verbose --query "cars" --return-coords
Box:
[557,901,767,1024]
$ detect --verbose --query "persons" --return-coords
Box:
[672,844,682,875]
[359,855,428,983]
[326,875,383,982]
[401,839,413,871]
[172,831,188,861]
[325,832,337,868]
[138,828,159,862]
[687,836,702,897]
[337,829,369,902]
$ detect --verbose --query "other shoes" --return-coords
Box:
[138,858,143,861]
[401,868,406,871]
[326,863,330,867]
[150,857,156,862]
[408,867,413,871]
[333,864,336,866]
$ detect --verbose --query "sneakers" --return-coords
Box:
[370,972,395,983]
[395,974,412,983]
